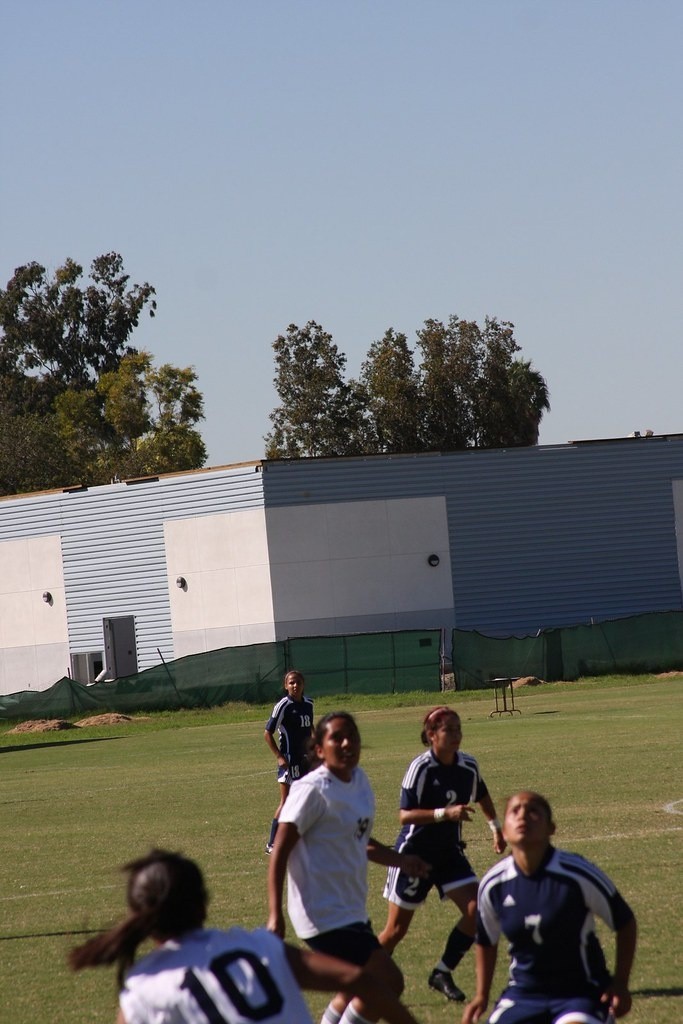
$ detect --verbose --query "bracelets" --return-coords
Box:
[434,807,446,821]
[487,818,502,832]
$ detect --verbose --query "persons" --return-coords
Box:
[376,705,508,999]
[267,710,431,1024]
[462,790,637,1024]
[263,670,322,855]
[65,849,378,1024]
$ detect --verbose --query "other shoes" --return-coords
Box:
[265,843,276,856]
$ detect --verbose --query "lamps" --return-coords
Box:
[427,554,440,566]
[43,591,52,602]
[176,577,186,588]
[255,465,264,473]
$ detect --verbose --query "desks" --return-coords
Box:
[486,678,522,717]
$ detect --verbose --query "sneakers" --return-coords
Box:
[428,967,465,1001]
